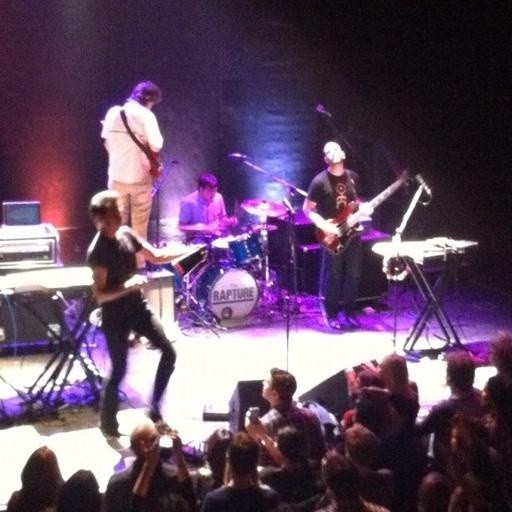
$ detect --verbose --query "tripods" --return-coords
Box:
[177,274,320,337]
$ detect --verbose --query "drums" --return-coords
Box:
[209,236,233,261]
[228,231,264,266]
[197,262,259,321]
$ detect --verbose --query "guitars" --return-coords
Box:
[314,171,408,256]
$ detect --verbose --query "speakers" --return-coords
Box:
[298,360,379,422]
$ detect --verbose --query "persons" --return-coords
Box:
[302,140,374,330]
[86,191,208,437]
[5,330,511,512]
[177,173,239,246]
[99,80,164,271]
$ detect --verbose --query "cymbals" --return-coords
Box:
[241,199,288,218]
[182,223,216,231]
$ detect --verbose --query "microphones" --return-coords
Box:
[226,153,247,161]
[416,173,431,195]
